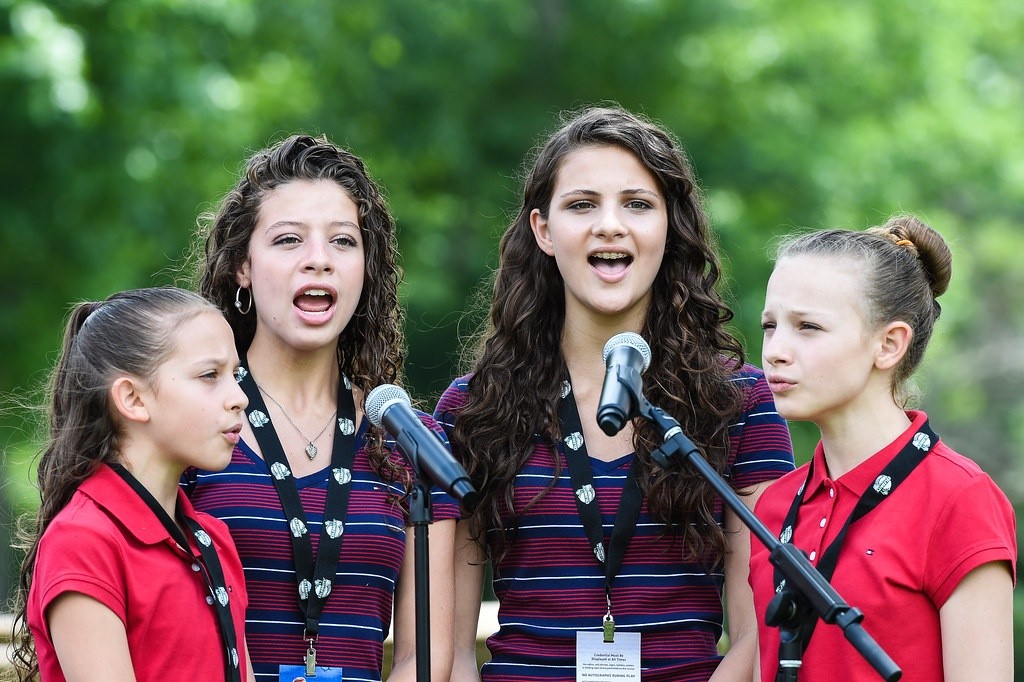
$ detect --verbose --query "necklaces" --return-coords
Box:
[252,378,338,461]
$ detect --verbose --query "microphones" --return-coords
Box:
[596,330,652,437]
[363,382,478,508]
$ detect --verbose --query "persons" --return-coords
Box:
[166,132,461,682]
[713,215,1015,682]
[430,103,795,682]
[0,286,258,682]
